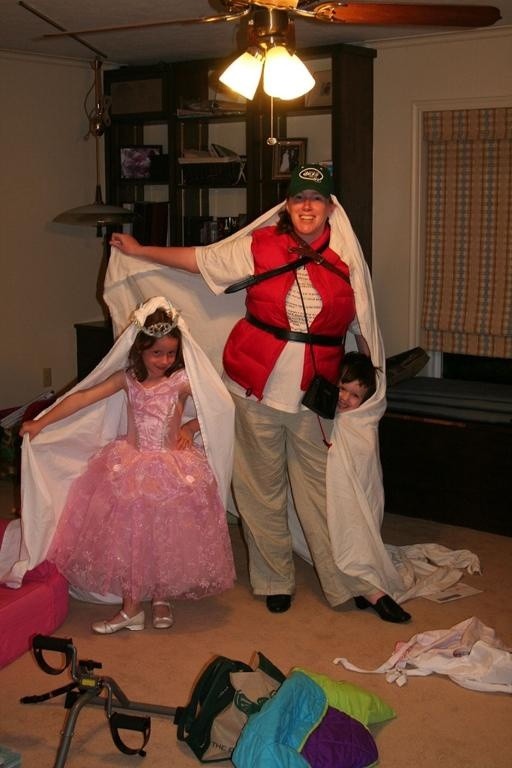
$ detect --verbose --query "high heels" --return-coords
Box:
[90,608,144,635]
[353,593,411,624]
[151,601,174,630]
[266,593,291,614]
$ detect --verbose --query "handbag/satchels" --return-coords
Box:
[302,373,340,420]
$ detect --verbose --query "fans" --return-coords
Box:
[41,0,501,40]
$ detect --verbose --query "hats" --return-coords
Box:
[287,164,333,198]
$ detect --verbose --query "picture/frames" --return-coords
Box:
[271,139,306,181]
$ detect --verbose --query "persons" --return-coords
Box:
[19,306,238,635]
[333,347,384,414]
[288,148,297,171]
[108,161,413,625]
[279,150,289,174]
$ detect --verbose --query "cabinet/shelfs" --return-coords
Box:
[74,322,115,382]
[103,44,378,282]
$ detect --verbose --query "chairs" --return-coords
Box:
[0,519,70,670]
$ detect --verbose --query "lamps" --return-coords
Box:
[51,58,140,237]
[216,32,318,105]
